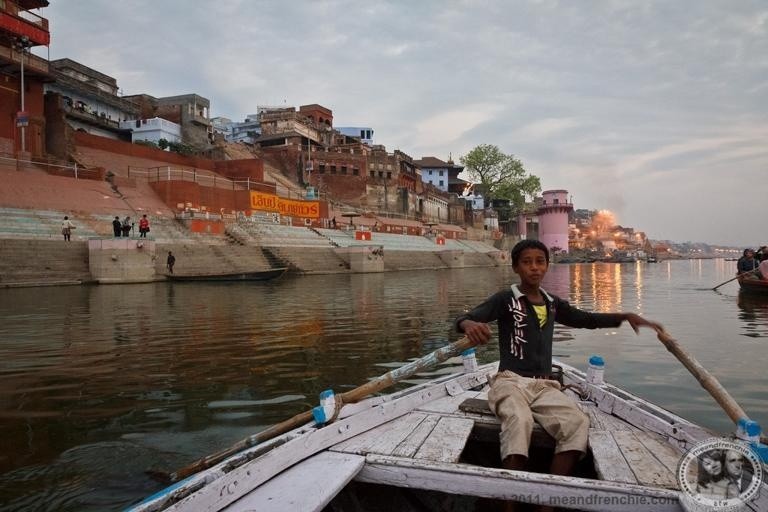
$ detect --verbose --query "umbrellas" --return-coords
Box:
[423,220,439,234]
[340,211,362,225]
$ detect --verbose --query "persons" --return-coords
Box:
[120,216,133,238]
[62,215,76,241]
[735,298,767,338]
[166,251,176,275]
[166,280,176,308]
[137,214,150,240]
[734,244,768,280]
[111,216,121,238]
[452,240,664,510]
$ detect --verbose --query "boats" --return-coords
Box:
[124,352,767,509]
[555,247,660,264]
[733,270,767,291]
